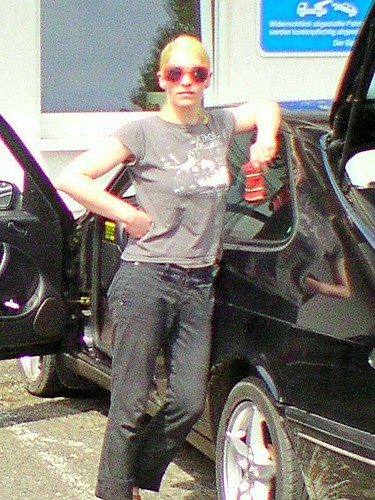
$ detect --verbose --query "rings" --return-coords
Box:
[265,153,271,157]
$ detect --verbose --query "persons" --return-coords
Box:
[228,138,353,326]
[56,34,283,500]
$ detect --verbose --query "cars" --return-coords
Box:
[0,0,375,500]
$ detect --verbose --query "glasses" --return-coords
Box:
[160,65,209,84]
